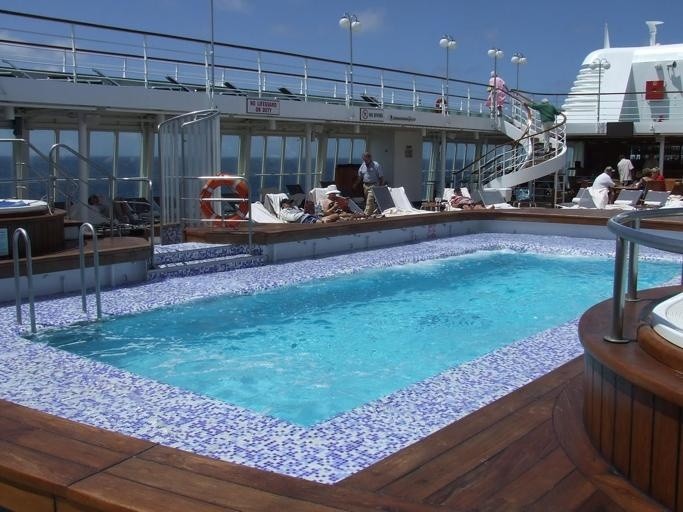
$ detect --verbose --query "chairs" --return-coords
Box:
[478,189,521,210]
[0,59,25,78]
[53,196,176,239]
[360,93,381,108]
[670,182,683,196]
[47,67,120,86]
[675,180,682,182]
[274,87,302,102]
[370,185,441,217]
[513,188,532,208]
[442,187,474,211]
[151,73,190,90]
[286,184,304,196]
[320,181,336,188]
[555,188,672,209]
[263,192,304,223]
[310,184,341,212]
[639,180,667,204]
[219,80,246,96]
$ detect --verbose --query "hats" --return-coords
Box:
[325,184,341,195]
[605,166,616,172]
[282,198,294,203]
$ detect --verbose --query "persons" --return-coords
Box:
[358,152,384,216]
[487,71,509,118]
[280,199,339,224]
[589,154,665,208]
[88,195,137,224]
[322,184,385,221]
[450,188,495,210]
[524,98,561,154]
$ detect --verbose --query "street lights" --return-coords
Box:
[587,57,612,124]
[438,32,457,108]
[486,47,504,118]
[509,48,528,96]
[337,11,362,105]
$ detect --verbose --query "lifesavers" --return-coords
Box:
[199,173,249,228]
